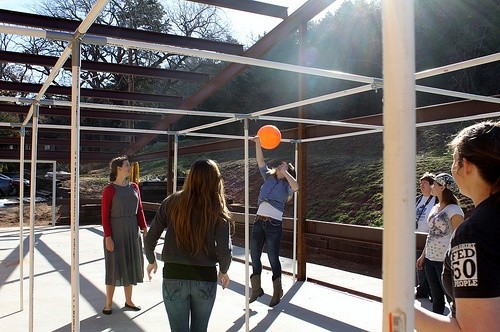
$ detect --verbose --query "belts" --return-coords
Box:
[254,214,273,222]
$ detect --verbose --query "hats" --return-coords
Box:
[430,173,457,193]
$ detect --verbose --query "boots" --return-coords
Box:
[269,275,283,307]
[249,274,264,303]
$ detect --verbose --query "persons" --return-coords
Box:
[249,136,299,306]
[415,171,438,232]
[143,159,235,332]
[417,173,464,318]
[101,157,148,315]
[413,122,500,332]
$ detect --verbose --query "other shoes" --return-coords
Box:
[103,307,112,314]
[125,302,141,310]
[415,291,429,299]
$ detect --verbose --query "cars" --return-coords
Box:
[0,174,30,196]
